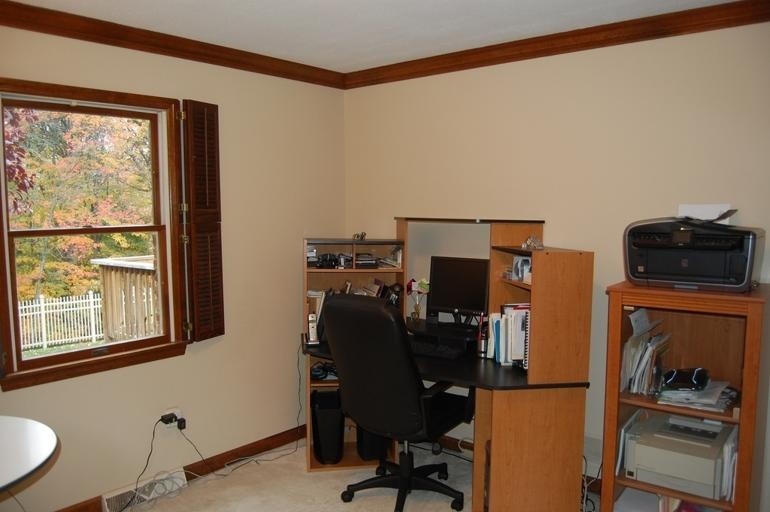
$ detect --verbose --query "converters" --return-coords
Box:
[161,413,177,424]
[178,418,185,429]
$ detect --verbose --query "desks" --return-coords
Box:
[1,411,63,512]
[306,339,582,509]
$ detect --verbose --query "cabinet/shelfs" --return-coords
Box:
[297,235,410,334]
[599,279,769,510]
[490,245,593,383]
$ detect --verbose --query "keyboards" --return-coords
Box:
[410,341,462,361]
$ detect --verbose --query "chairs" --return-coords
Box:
[319,291,473,509]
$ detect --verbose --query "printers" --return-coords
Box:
[623,217,765,292]
[624,414,740,501]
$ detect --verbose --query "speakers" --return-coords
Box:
[426,294,438,325]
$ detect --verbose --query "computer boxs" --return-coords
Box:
[416,323,477,353]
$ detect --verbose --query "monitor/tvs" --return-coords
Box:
[428,256,489,329]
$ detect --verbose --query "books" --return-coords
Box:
[486,303,530,371]
[614,408,643,477]
[723,425,739,506]
[620,319,674,397]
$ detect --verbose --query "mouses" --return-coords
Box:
[460,353,473,362]
